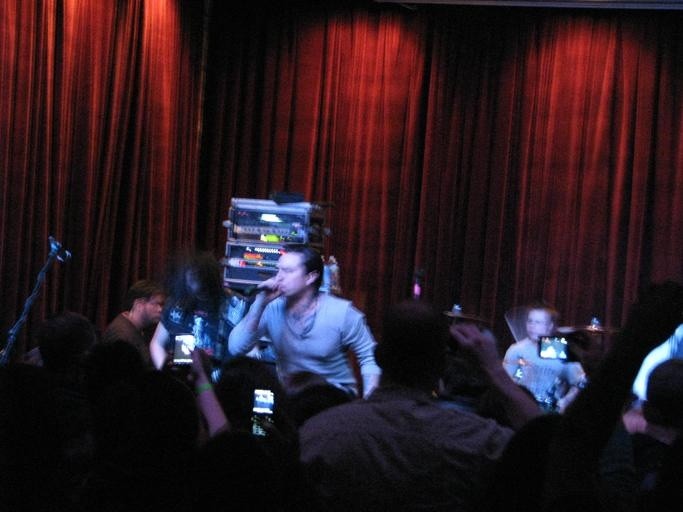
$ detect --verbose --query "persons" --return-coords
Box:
[102,281,165,360]
[150,263,226,371]
[1,292,682,512]
[226,247,381,403]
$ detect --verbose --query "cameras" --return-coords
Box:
[538,333,590,363]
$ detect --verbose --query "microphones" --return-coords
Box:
[49,235,72,261]
[245,282,278,296]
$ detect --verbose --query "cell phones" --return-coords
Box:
[172,333,194,366]
[251,385,278,441]
